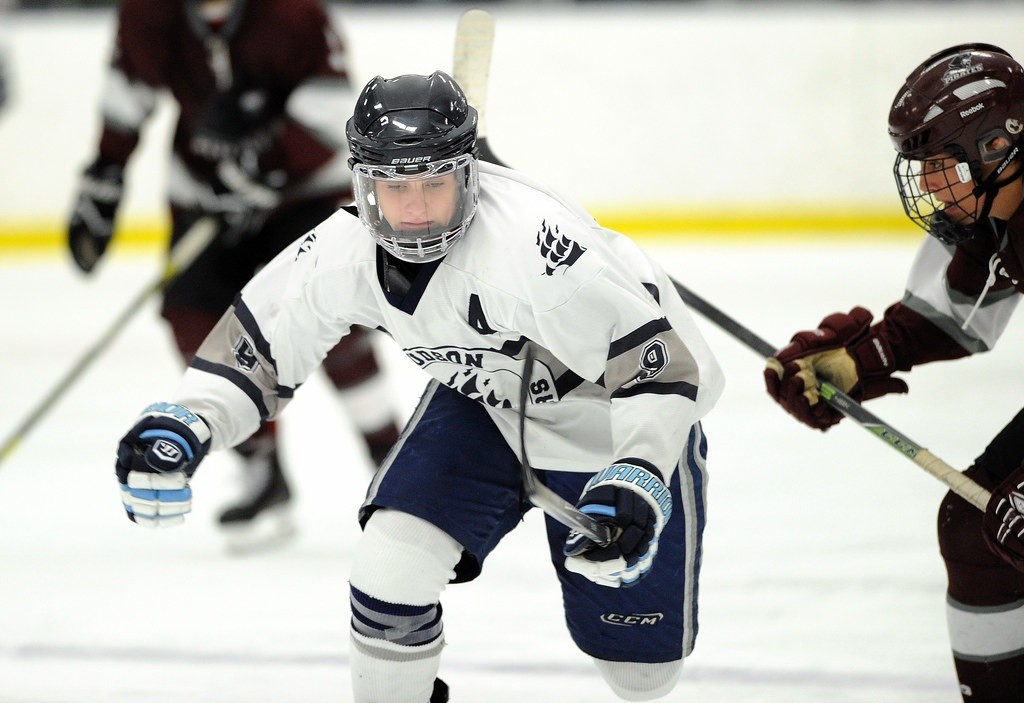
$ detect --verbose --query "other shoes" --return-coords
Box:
[219,422,290,522]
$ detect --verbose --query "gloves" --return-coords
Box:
[115,401,213,528]
[563,457,673,588]
[763,306,912,431]
[200,133,296,230]
[70,166,123,271]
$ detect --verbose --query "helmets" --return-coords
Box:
[888,43,1024,246]
[346,71,479,182]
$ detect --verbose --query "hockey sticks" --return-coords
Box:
[1,219,214,464]
[516,343,611,541]
[451,8,993,513]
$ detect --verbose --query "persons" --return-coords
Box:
[66,0,725,703]
[763,42,1024,703]
